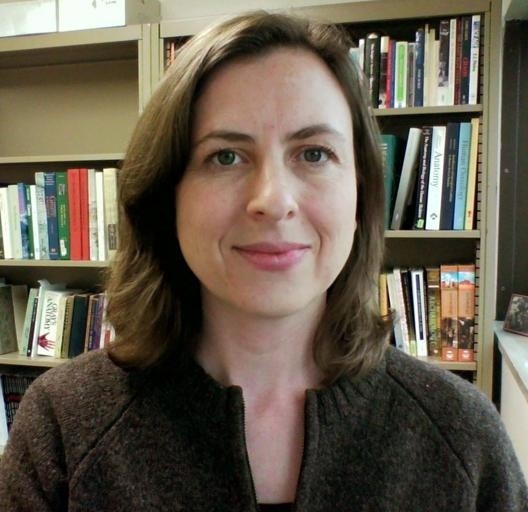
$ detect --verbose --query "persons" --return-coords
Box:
[1,10,528,512]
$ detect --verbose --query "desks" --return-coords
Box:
[493,318,527,489]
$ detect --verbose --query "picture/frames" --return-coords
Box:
[502,292,528,336]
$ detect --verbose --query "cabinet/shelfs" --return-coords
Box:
[1,1,504,448]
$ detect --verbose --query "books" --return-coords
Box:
[379,114,481,230]
[0,373,40,436]
[0,167,126,261]
[0,280,125,360]
[349,15,481,109]
[378,263,476,362]
